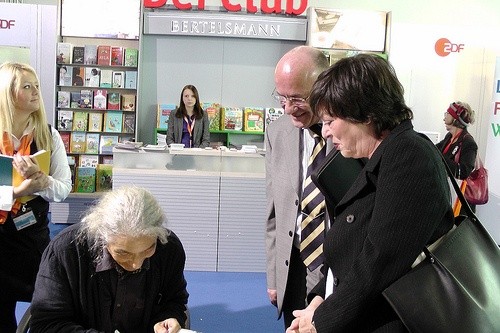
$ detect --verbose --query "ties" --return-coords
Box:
[299,123,327,272]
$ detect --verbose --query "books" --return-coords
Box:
[0,149,51,203]
[58,43,136,154]
[68,155,113,192]
[116,140,266,154]
[200,102,285,132]
[156,104,177,147]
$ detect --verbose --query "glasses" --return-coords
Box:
[271,88,311,106]
[444,112,450,118]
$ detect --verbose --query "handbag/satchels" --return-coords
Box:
[454,133,489,205]
[381,132,500,333]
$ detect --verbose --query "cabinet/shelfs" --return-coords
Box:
[47,0,307,272]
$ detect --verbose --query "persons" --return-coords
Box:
[434,102,477,216]
[264,46,335,333]
[28,188,190,333]
[285,54,455,333]
[0,61,72,333]
[166,85,211,149]
